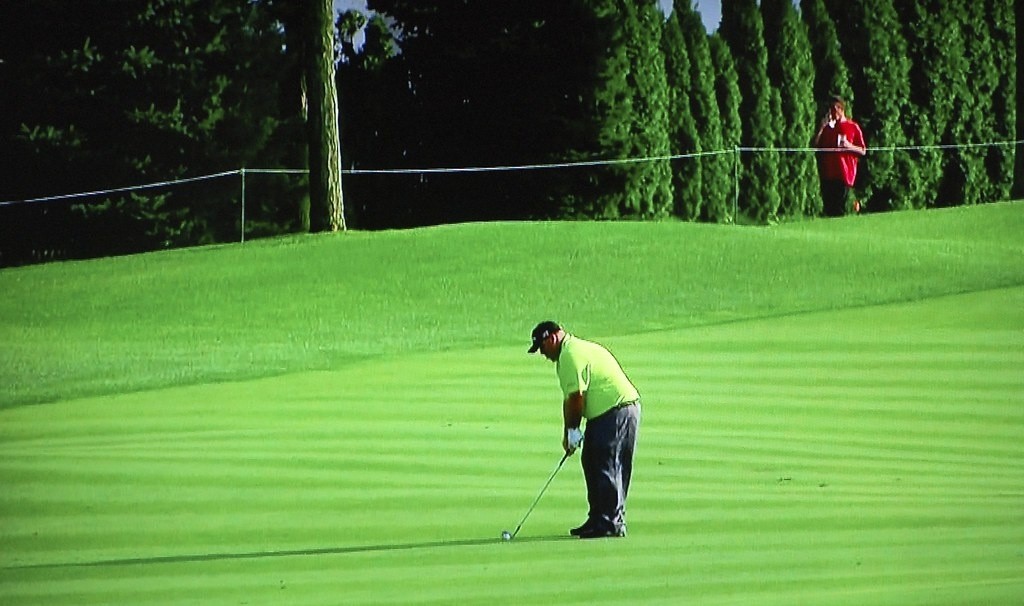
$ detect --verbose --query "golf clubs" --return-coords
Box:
[501,448,569,541]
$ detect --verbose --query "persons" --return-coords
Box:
[814,95,866,217]
[527,320,641,539]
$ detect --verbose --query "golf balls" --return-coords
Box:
[503,533,510,541]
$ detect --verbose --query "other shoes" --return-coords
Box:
[570,519,593,535]
[579,523,623,538]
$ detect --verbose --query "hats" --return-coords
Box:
[527,321,560,353]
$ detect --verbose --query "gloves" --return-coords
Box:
[568,427,582,450]
